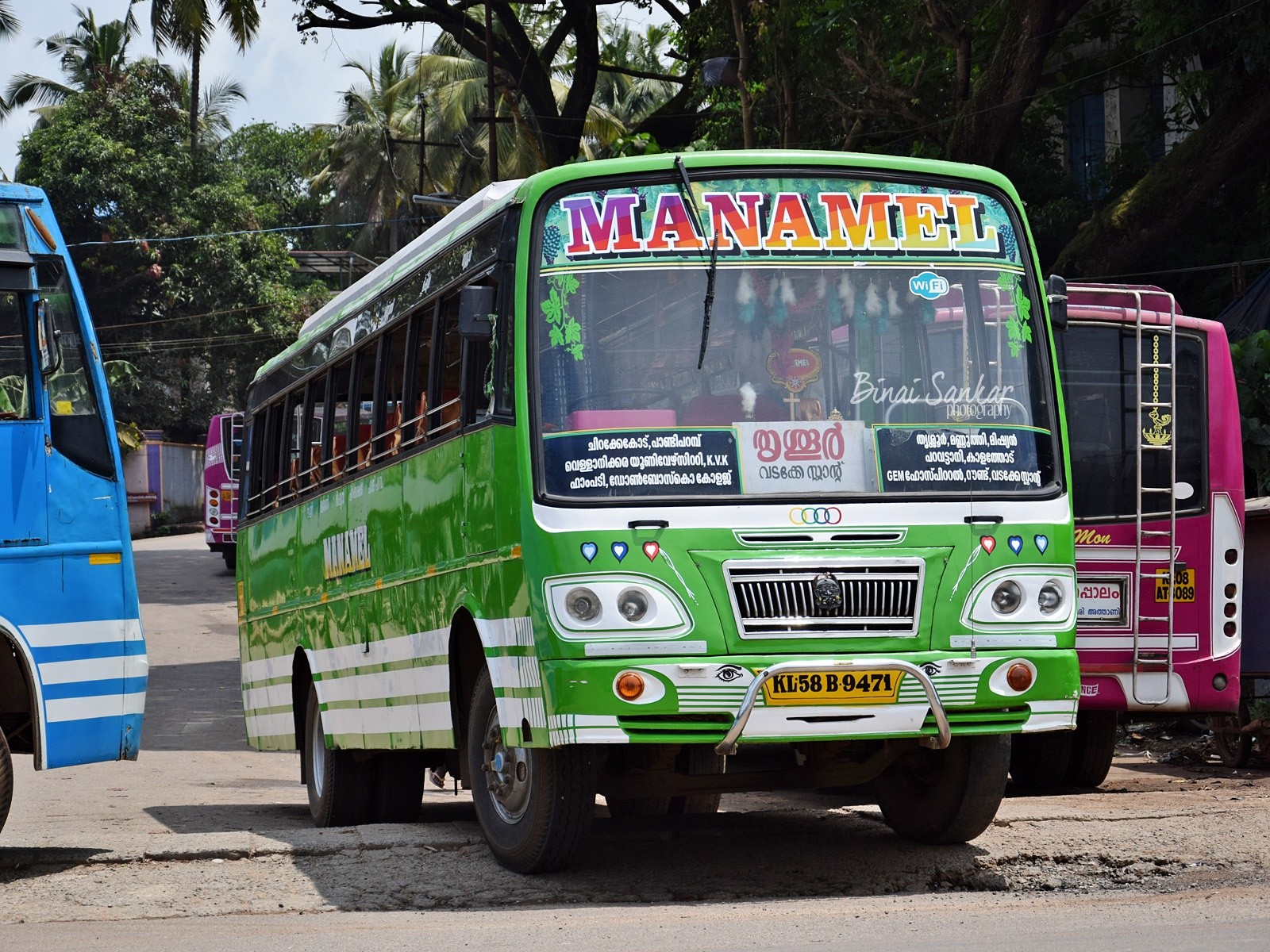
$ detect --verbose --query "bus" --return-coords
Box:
[0,176,154,833]
[231,139,1088,876]
[361,401,403,418]
[643,274,1246,791]
[202,401,373,572]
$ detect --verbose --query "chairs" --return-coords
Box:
[287,374,824,503]
